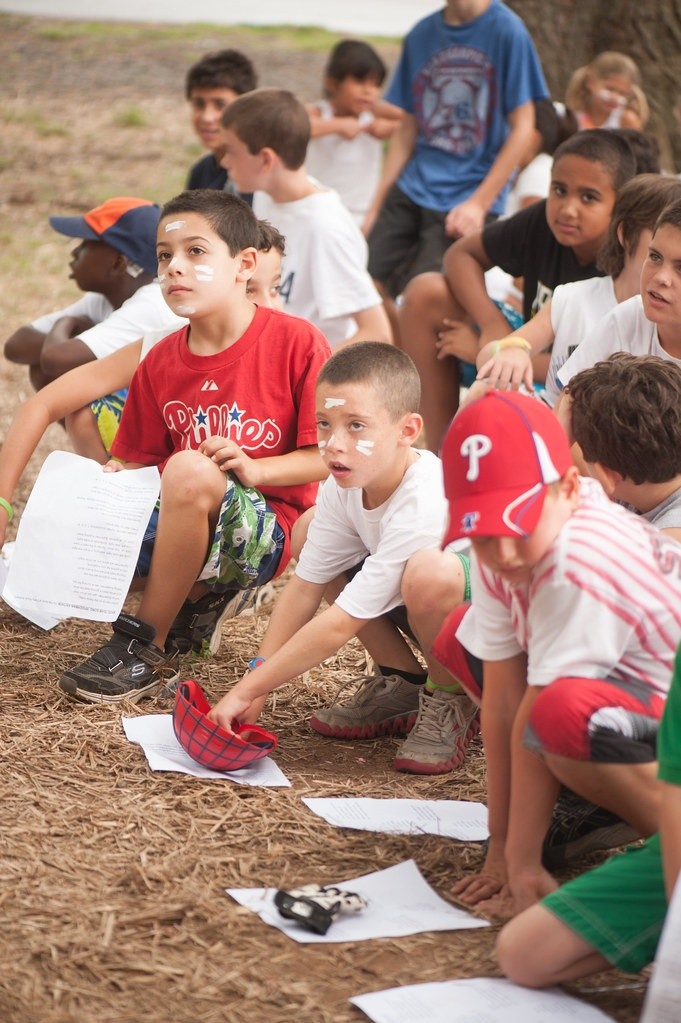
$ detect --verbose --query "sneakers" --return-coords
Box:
[394,684,480,774]
[310,664,425,739]
[480,785,643,867]
[164,580,258,658]
[58,613,180,707]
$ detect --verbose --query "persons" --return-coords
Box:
[484,100,560,314]
[399,128,638,456]
[0,221,286,551]
[365,1,551,456]
[221,89,391,352]
[496,641,681,987]
[208,341,483,773]
[186,48,258,211]
[306,40,404,224]
[567,353,681,545]
[568,51,644,132]
[4,196,170,435]
[60,191,332,703]
[455,173,681,409]
[559,196,681,446]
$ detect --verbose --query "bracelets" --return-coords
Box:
[248,657,265,669]
[0,498,14,522]
[495,337,531,351]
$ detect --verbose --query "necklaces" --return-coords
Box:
[430,387,681,920]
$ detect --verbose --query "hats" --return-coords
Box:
[441,389,574,549]
[173,680,278,771]
[49,196,162,276]
